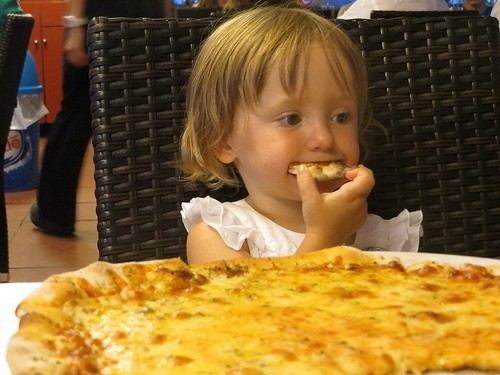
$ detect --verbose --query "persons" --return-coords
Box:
[176,7,424,265]
[29,0,167,234]
[163,0,500,20]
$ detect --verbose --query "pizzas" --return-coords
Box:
[287,160,358,181]
[6,245,500,375]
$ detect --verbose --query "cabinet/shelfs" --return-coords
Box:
[18,0,68,124]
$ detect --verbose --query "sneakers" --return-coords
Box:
[31,202,75,237]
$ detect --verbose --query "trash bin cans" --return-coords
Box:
[3,51,49,193]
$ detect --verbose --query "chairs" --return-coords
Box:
[85,12,500,265]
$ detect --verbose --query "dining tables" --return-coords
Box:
[0,251,500,375]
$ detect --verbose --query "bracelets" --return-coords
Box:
[62,16,89,28]
